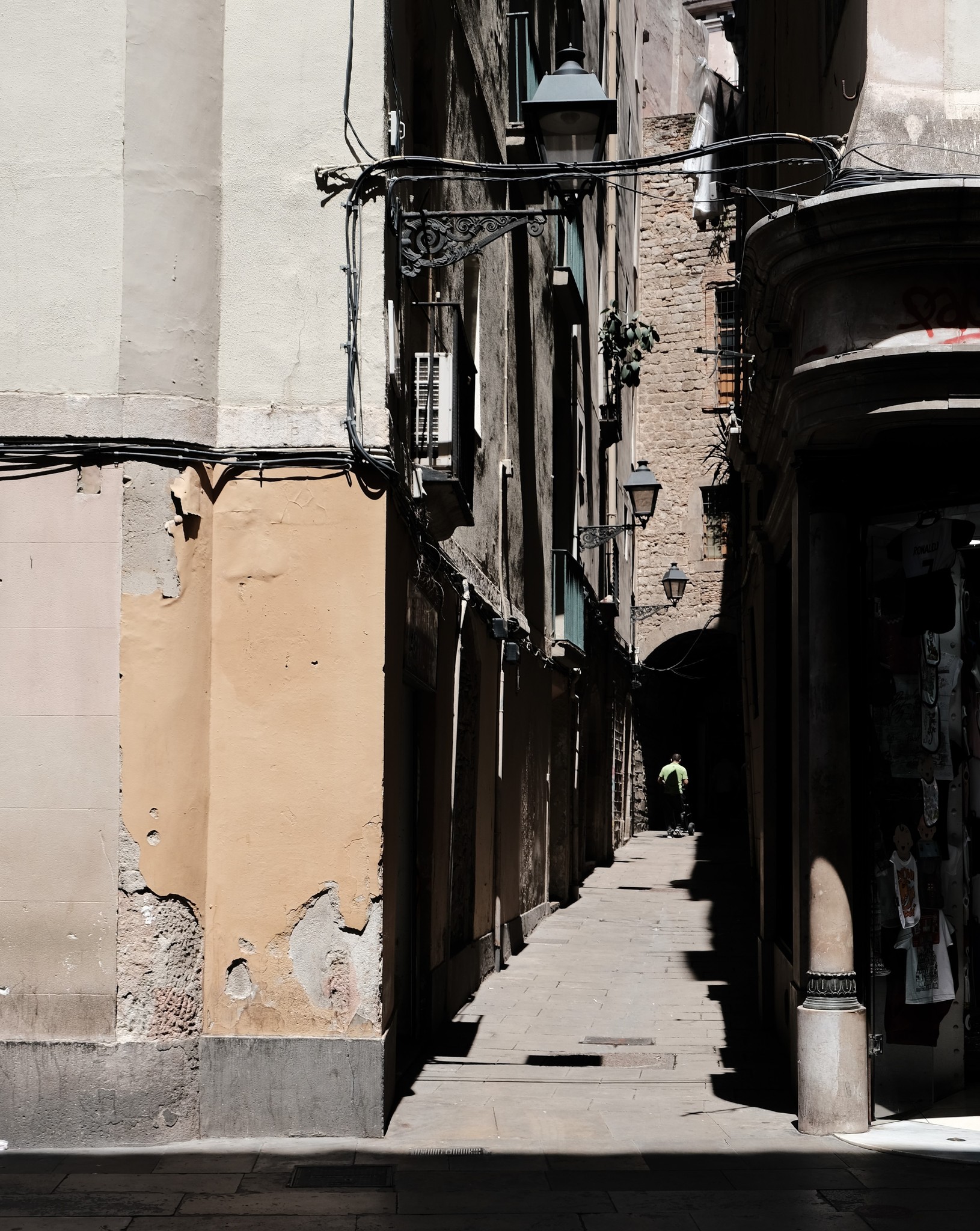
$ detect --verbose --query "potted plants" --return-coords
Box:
[597,298,660,422]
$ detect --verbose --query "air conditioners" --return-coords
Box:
[411,352,454,468]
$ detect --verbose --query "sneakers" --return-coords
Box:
[667,831,675,838]
[675,827,685,837]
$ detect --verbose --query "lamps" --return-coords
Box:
[576,460,663,566]
[630,563,689,623]
[399,42,617,279]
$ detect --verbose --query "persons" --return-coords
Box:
[657,753,690,838]
